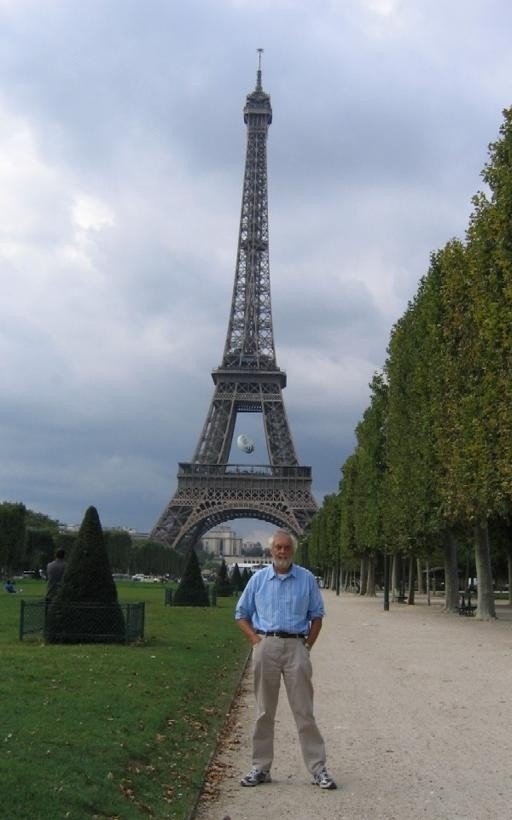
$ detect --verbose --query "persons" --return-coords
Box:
[47,548,68,597]
[234,530,339,789]
[4,579,19,593]
[39,567,47,584]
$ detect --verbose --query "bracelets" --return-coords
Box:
[305,642,311,650]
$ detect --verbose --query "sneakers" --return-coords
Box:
[311,767,337,790]
[240,767,272,787]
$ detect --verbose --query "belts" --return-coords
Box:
[256,630,305,639]
